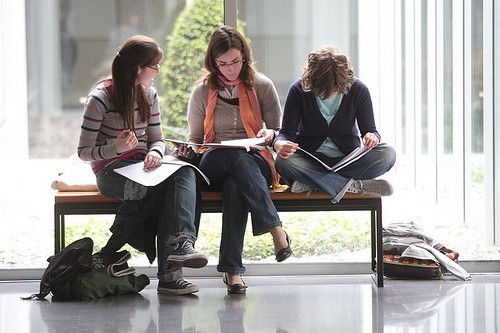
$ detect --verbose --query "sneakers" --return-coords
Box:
[290,180,318,197]
[354,179,393,197]
[157,277,199,295]
[167,240,208,268]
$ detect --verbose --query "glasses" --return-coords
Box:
[146,64,160,72]
[214,57,245,69]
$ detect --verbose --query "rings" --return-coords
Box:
[152,159,157,162]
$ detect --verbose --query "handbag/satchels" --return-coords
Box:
[20,237,94,302]
[375,243,459,280]
[68,250,151,302]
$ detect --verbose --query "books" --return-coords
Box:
[113,155,210,188]
[295,145,374,173]
[165,137,267,153]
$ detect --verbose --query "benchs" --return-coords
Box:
[54,190,385,289]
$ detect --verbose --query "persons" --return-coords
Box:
[272,46,397,203]
[175,25,292,294]
[78,36,209,295]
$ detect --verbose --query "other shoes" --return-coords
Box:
[222,271,249,294]
[274,229,293,263]
